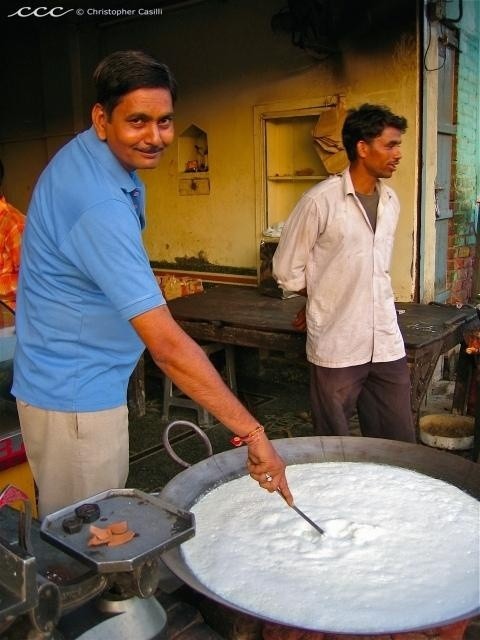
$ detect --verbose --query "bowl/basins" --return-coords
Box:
[419,413,475,450]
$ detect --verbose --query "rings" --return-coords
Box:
[265,473,272,481]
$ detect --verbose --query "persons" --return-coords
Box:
[273,102,418,444]
[9,48,293,523]
[0,163,27,329]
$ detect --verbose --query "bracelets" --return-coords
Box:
[230,425,264,447]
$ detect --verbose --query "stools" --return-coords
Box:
[161,343,239,431]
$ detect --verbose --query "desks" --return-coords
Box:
[131,285,479,442]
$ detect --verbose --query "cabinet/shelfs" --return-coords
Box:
[261,105,331,245]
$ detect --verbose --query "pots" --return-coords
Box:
[154,436,479,635]
